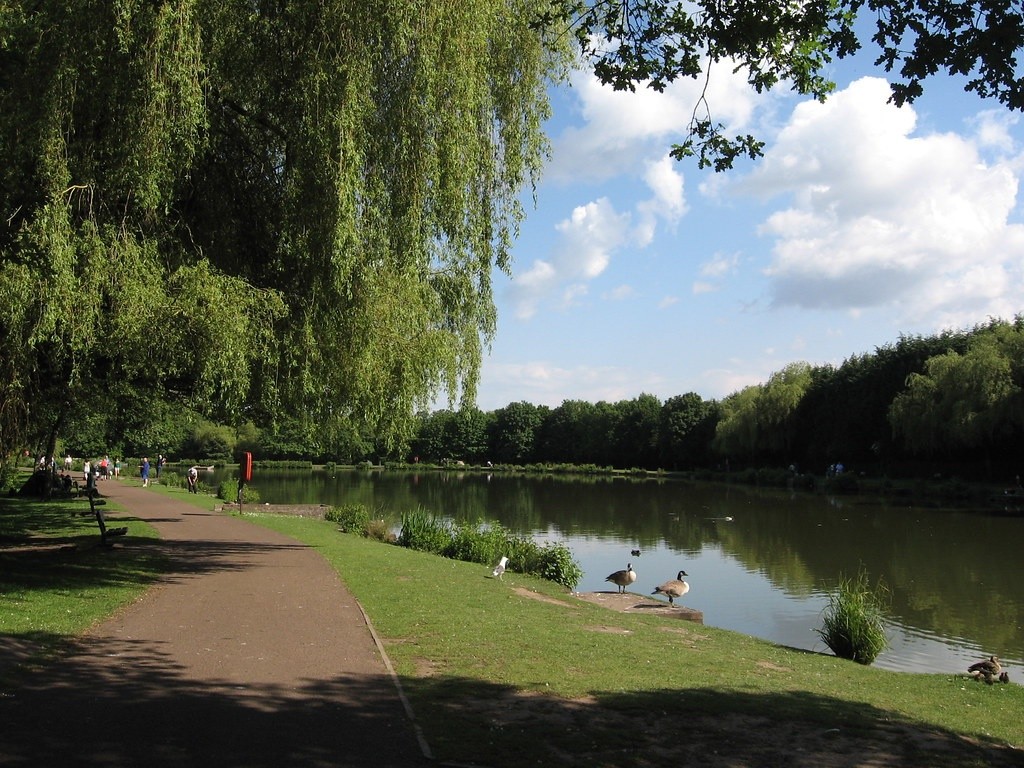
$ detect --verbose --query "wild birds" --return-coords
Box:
[968,655,1009,684]
[490,557,509,580]
[604,564,636,593]
[631,550,640,555]
[651,570,689,605]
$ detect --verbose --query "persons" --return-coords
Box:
[156,454,166,479]
[23,450,122,498]
[186,467,198,495]
[138,457,149,488]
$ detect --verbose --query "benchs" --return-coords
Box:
[89,495,107,514]
[96,511,128,547]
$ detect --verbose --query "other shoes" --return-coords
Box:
[189,492,198,494]
[143,484,147,487]
